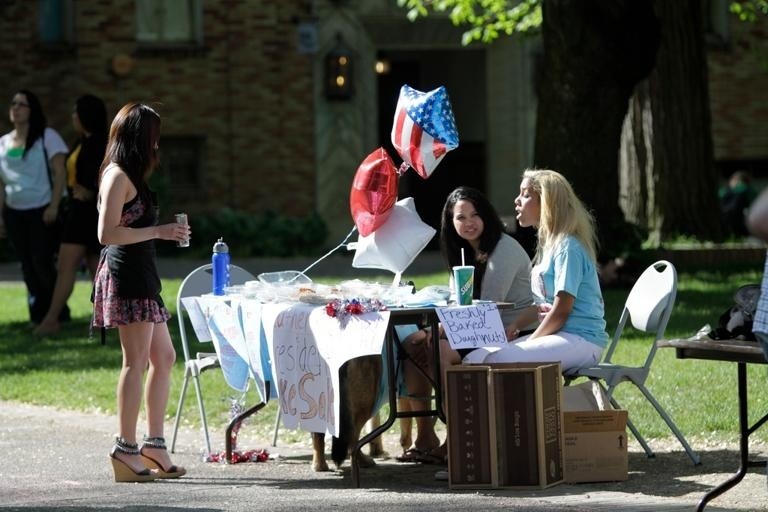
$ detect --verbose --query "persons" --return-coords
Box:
[0,86,72,329]
[460,168,609,372]
[746,190,768,339]
[32,93,109,337]
[90,102,191,483]
[397,184,538,466]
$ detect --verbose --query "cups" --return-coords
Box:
[452,266,474,304]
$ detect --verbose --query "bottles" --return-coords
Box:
[174,215,189,248]
[213,238,230,295]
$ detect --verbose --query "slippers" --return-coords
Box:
[395,448,423,462]
[416,448,444,466]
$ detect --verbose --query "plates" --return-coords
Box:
[258,271,312,289]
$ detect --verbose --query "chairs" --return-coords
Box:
[561,260,702,467]
[172,263,283,454]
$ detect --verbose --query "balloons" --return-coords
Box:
[390,83,459,179]
[351,197,436,273]
[349,146,398,237]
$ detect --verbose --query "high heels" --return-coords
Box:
[139,435,186,478]
[109,437,161,481]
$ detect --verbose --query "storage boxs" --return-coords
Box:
[561,409,629,485]
[445,361,563,490]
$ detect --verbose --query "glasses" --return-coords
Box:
[10,100,29,108]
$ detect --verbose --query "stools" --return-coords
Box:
[675,340,768,512]
